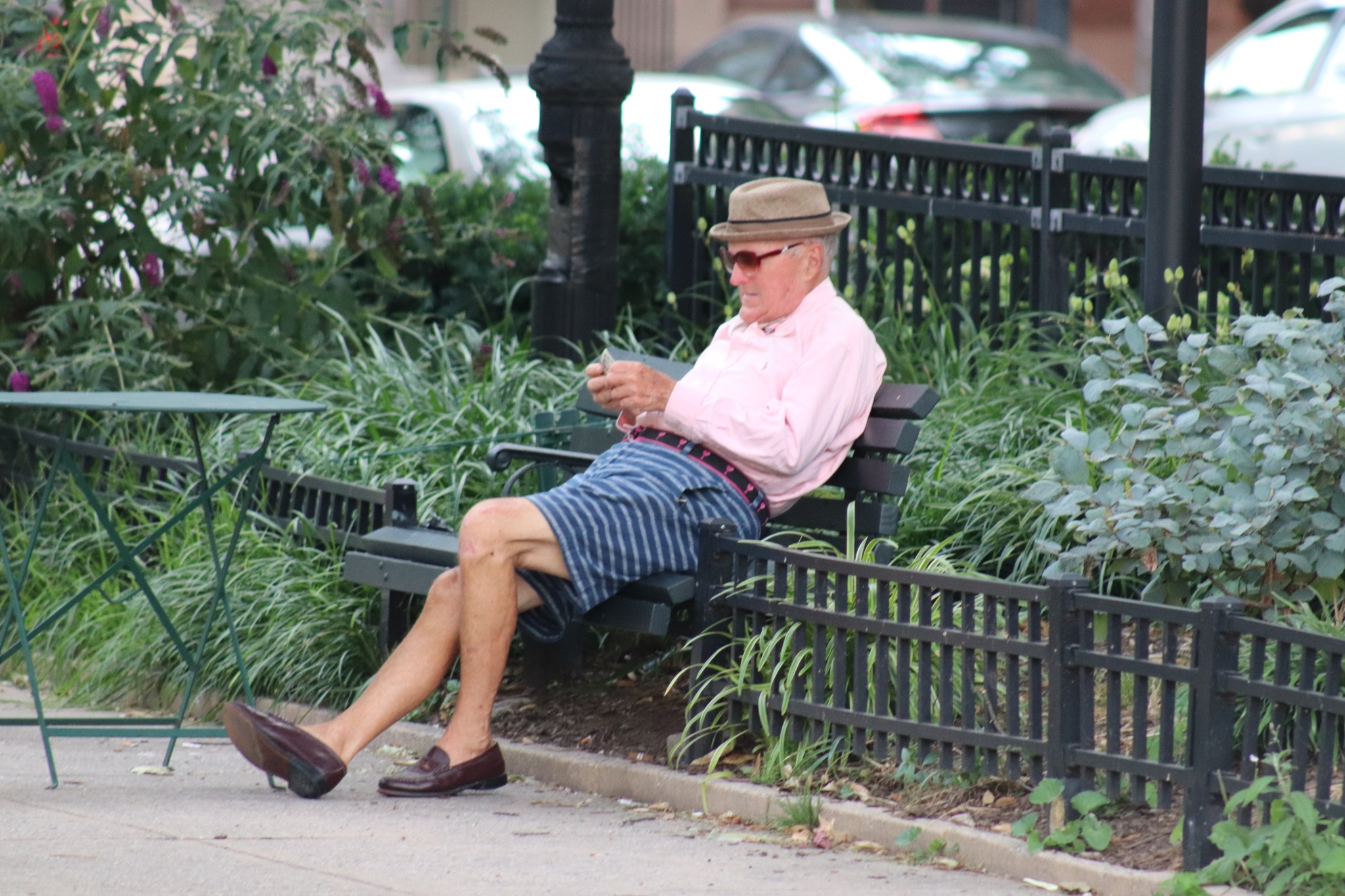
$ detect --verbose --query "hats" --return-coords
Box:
[709,176,852,242]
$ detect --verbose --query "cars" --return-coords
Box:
[1066,0,1345,226]
[342,68,805,189]
[677,9,1123,151]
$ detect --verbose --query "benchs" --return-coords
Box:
[347,349,937,699]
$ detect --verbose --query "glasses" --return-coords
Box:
[719,242,805,275]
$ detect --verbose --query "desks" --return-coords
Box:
[0,392,330,792]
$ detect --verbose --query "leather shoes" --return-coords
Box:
[221,701,347,800]
[378,739,508,799]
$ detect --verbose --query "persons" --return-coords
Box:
[222,176,889,797]
